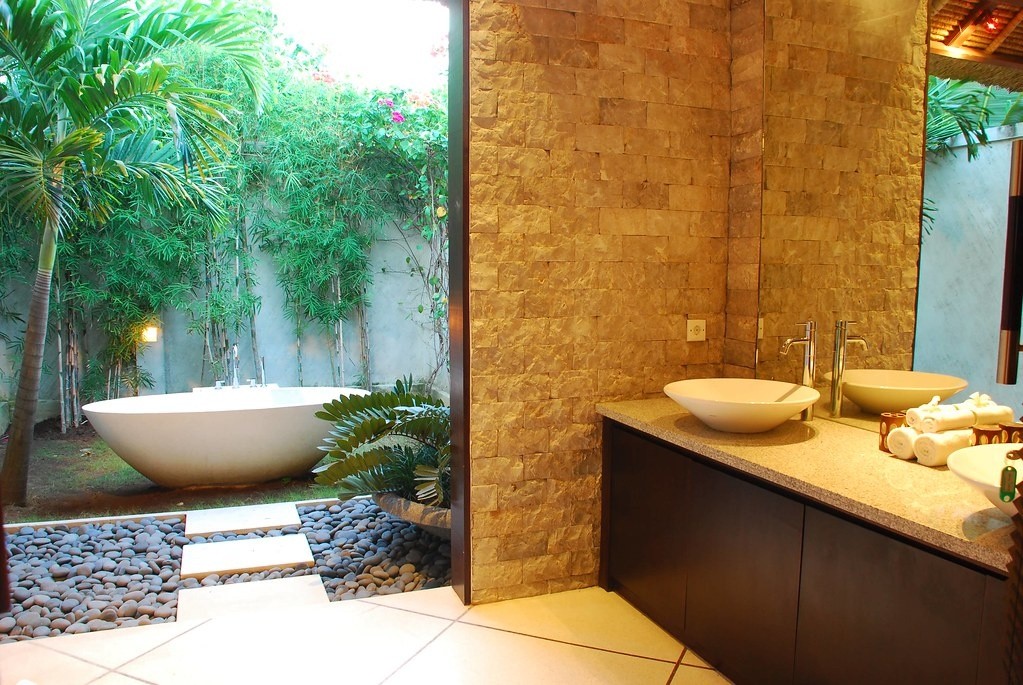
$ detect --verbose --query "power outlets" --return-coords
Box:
[687,320,706,341]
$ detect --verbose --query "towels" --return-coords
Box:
[887,395,976,469]
[951,392,1016,425]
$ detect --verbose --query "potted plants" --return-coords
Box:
[312,373,451,540]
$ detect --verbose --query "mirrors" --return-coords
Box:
[753,0,1023,445]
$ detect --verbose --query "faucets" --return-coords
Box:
[829,319,868,418]
[231,343,241,386]
[780,320,818,422]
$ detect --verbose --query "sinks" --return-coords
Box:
[947,442,1023,518]
[824,367,969,415]
[664,377,821,434]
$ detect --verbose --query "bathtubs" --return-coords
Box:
[80,385,372,487]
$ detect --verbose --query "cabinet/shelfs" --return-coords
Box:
[602,416,1008,685]
